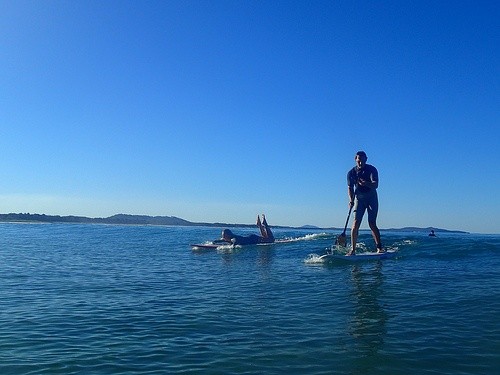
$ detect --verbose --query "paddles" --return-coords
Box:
[334,183,359,248]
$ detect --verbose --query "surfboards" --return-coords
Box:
[190,241,231,248]
[321,246,400,262]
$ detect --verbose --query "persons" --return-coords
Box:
[220,214,275,244]
[429,230,436,237]
[347,151,384,255]
